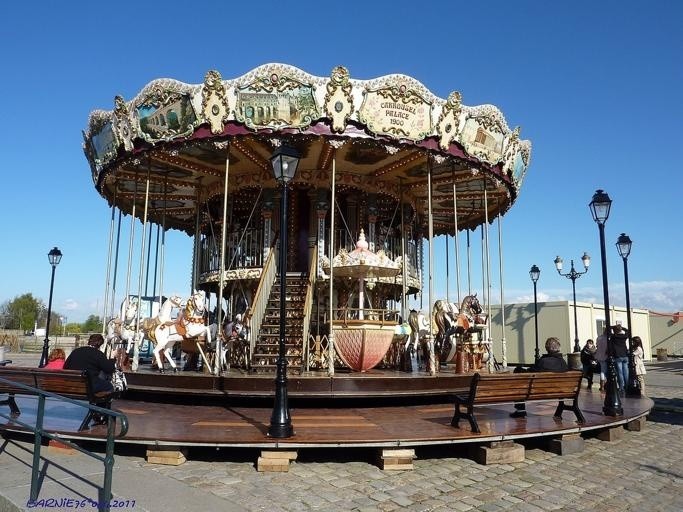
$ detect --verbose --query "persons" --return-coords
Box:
[597,328,608,392]
[580,339,599,390]
[605,325,631,394]
[628,336,646,395]
[508,337,569,419]
[39,348,64,371]
[61,334,115,426]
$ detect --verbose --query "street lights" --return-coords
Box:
[588,187,623,417]
[58,315,63,338]
[528,264,542,368]
[610,232,641,398]
[552,252,592,346]
[267,142,299,438]
[38,246,65,368]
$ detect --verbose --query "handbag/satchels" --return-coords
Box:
[109,369,128,395]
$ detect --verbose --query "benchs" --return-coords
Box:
[452,370,586,433]
[0,360,114,431]
